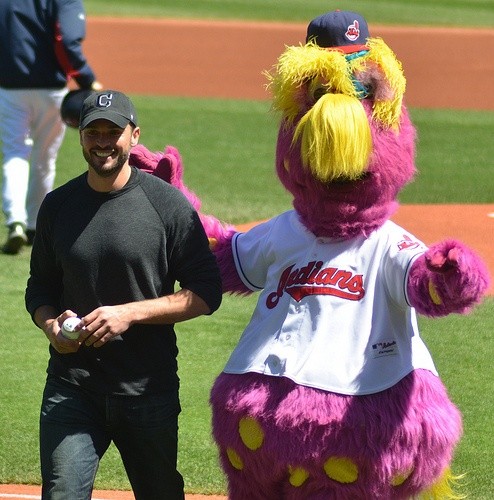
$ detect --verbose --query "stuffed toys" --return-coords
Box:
[128,11,487,500]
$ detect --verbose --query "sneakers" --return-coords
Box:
[1,222,27,255]
[25,230,35,245]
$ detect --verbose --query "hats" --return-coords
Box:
[306,8,370,54]
[79,90,137,128]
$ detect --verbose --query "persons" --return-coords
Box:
[24,89,222,500]
[1,0,102,255]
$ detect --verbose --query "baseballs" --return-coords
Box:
[61,317,85,339]
